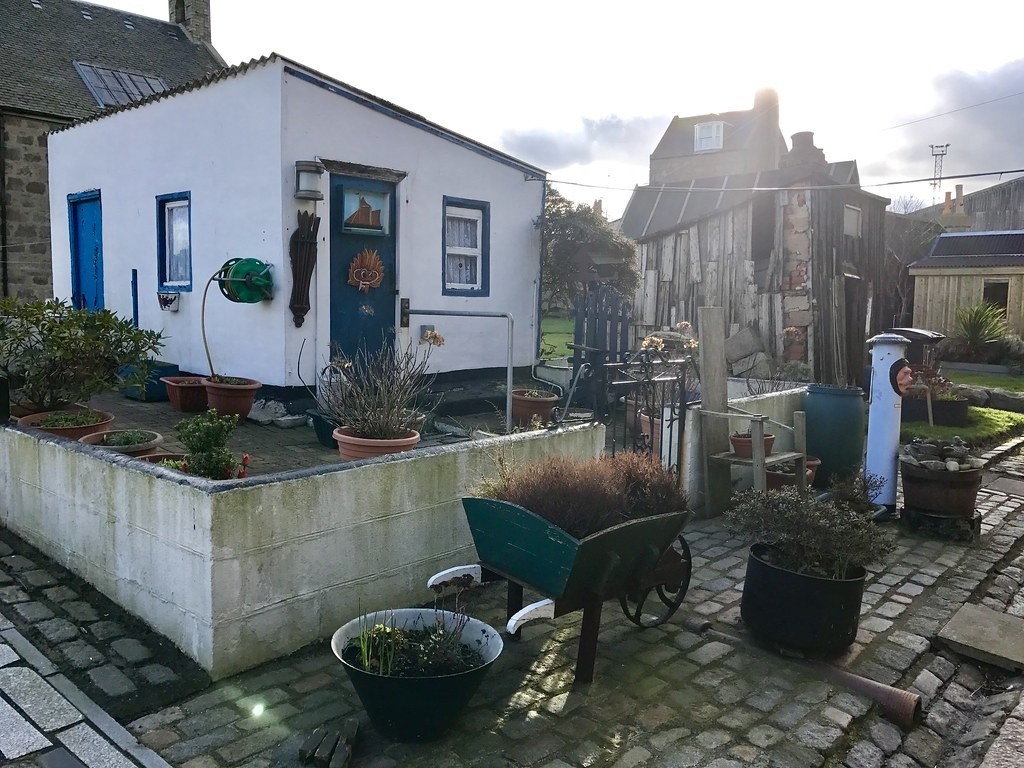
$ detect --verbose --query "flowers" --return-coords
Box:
[297,324,444,440]
[171,408,250,479]
[638,321,702,418]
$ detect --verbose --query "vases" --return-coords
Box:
[636,405,681,455]
[135,454,189,463]
[306,409,426,461]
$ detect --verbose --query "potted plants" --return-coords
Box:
[931,391,968,428]
[619,363,679,438]
[729,346,898,657]
[900,436,986,516]
[0,288,262,463]
[900,394,928,423]
[331,573,503,740]
[944,298,1014,364]
[427,400,693,684]
[512,376,561,427]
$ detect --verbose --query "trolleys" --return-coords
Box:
[426,496,697,700]
